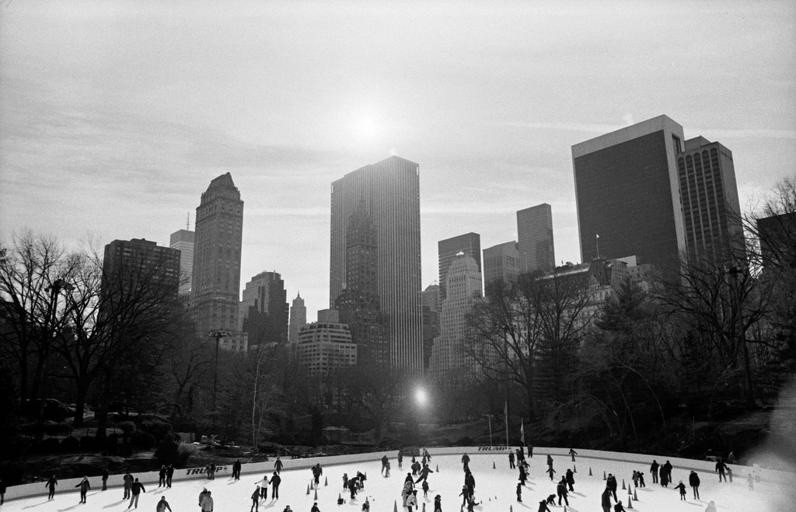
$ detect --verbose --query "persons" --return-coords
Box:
[99,467,112,491]
[248,444,579,510]
[74,474,92,505]
[714,458,734,482]
[703,499,720,510]
[0,478,6,505]
[126,476,146,509]
[155,495,173,511]
[230,457,243,479]
[158,462,177,488]
[196,486,214,511]
[745,473,756,491]
[204,460,220,480]
[44,473,59,500]
[121,469,135,499]
[598,459,702,511]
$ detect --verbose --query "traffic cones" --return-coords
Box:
[324,476,328,486]
[436,464,440,473]
[306,483,311,495]
[310,480,315,490]
[394,499,399,512]
[572,464,639,508]
[313,489,319,500]
[493,461,497,469]
[509,505,513,512]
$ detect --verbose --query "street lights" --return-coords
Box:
[495,319,520,441]
[210,327,231,450]
[35,273,77,472]
